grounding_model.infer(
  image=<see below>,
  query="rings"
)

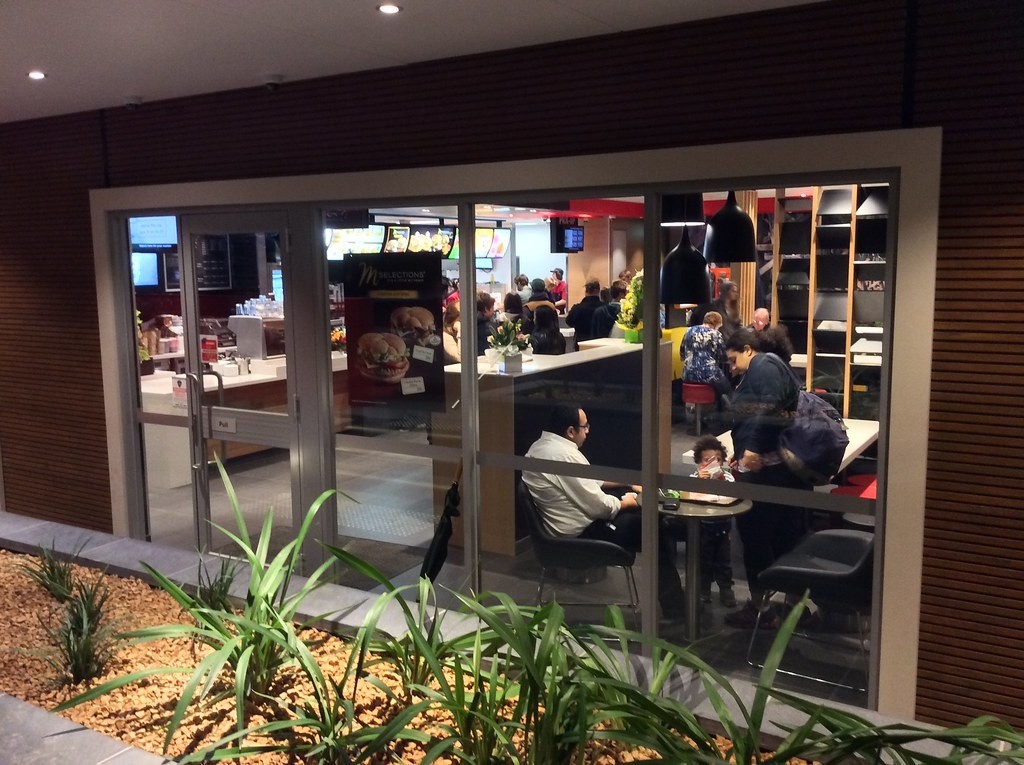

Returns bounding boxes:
[751,460,757,464]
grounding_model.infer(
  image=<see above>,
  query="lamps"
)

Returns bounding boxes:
[661,224,709,304]
[703,189,757,262]
[661,193,705,227]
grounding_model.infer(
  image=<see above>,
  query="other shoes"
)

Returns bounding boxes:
[720,588,739,608]
[698,585,714,601]
[768,598,813,627]
[663,598,705,620]
[725,597,777,630]
[687,427,712,436]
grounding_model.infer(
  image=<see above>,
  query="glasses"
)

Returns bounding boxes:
[752,319,768,325]
[578,423,590,429]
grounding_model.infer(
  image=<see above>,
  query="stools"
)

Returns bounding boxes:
[681,384,716,436]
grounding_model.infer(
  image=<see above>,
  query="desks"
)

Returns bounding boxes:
[636,489,754,644]
[432,338,673,557]
[682,417,880,486]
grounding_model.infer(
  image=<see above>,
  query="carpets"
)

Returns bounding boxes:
[214,523,431,592]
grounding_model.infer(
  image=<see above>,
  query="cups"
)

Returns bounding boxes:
[141,326,179,354]
[235,294,283,318]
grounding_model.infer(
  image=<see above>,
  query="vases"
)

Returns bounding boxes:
[624,331,644,343]
[485,346,534,364]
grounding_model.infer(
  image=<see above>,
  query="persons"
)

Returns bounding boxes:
[521,402,704,620]
[680,280,836,630]
[439,267,632,363]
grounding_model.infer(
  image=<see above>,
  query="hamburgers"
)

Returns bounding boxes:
[357,332,412,384]
[390,305,435,346]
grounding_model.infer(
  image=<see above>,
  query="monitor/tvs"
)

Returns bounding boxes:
[129,215,178,293]
[563,226,584,249]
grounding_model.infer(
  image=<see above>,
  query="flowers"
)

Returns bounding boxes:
[487,318,530,353]
[614,272,644,331]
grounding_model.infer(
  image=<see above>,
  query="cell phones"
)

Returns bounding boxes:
[663,498,680,510]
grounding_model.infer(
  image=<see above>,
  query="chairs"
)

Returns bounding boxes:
[747,467,879,693]
[516,482,640,643]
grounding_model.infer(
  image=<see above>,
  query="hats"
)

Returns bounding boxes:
[529,278,545,292]
[550,268,563,276]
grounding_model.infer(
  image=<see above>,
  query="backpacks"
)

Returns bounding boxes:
[748,350,849,487]
